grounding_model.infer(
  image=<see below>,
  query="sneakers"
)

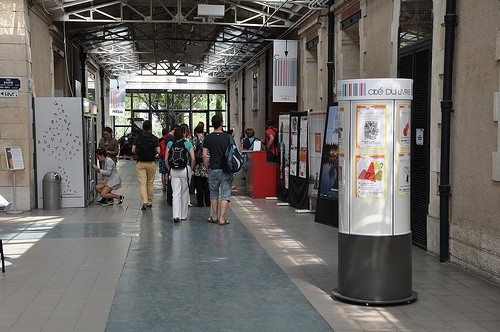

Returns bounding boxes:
[102,199,114,206]
[117,195,124,206]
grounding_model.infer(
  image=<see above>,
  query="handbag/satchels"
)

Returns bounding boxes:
[195,140,203,165]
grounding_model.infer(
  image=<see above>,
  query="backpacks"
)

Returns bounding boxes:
[243,137,256,150]
[138,133,155,161]
[168,139,187,169]
[225,134,244,173]
[272,128,278,145]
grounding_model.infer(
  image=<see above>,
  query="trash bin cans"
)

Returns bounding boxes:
[43,171,61,210]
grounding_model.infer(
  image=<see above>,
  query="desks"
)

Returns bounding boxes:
[246,163,277,199]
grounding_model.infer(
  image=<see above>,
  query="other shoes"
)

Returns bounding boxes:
[141,202,152,210]
[189,203,193,207]
[181,218,187,220]
[174,218,180,223]
[97,198,108,204]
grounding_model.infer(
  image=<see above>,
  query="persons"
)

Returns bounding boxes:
[165,126,196,222]
[131,120,160,211]
[158,117,279,206]
[202,114,237,226]
[92,149,125,206]
[96,127,118,202]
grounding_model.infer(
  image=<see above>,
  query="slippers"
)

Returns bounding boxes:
[219,220,229,225]
[207,216,218,223]
